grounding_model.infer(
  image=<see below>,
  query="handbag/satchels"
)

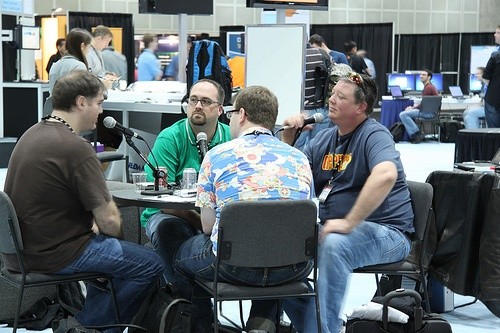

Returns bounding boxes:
[129,281,197,333]
[345,288,452,333]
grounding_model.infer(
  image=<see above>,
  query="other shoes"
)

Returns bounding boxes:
[413,135,424,143]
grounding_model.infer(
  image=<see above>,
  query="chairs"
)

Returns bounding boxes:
[413,96,441,141]
[353,180,434,314]
[45,96,130,183]
[0,191,122,333]
[189,199,322,333]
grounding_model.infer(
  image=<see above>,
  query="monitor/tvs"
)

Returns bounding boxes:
[388,73,415,91]
[389,86,403,97]
[415,73,443,93]
[449,86,463,97]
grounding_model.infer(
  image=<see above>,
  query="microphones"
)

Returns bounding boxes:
[103,116,144,140]
[197,131,208,160]
[277,113,324,132]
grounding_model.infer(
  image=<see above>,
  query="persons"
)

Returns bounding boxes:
[165,42,193,81]
[88,24,119,90]
[100,40,128,90]
[48,28,93,100]
[278,72,414,333]
[462,25,500,130]
[137,32,163,82]
[173,85,317,333]
[45,38,66,81]
[399,68,442,144]
[3,70,164,333]
[139,79,237,274]
[304,34,376,109]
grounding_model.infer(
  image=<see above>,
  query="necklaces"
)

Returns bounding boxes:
[41,114,76,133]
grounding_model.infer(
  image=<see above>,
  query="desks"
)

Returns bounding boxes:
[454,128,500,162]
[437,99,482,118]
[102,101,232,127]
[426,170,500,319]
[112,188,246,331]
[380,99,413,128]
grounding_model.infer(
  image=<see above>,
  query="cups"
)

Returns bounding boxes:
[132,173,147,193]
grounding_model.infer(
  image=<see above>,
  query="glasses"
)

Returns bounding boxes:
[187,97,219,107]
[343,71,368,103]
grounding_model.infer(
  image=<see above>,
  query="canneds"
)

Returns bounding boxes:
[154,167,167,191]
[183,167,197,189]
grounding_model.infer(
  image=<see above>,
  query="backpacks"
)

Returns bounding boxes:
[314,48,353,109]
[388,122,406,143]
[180,33,233,105]
